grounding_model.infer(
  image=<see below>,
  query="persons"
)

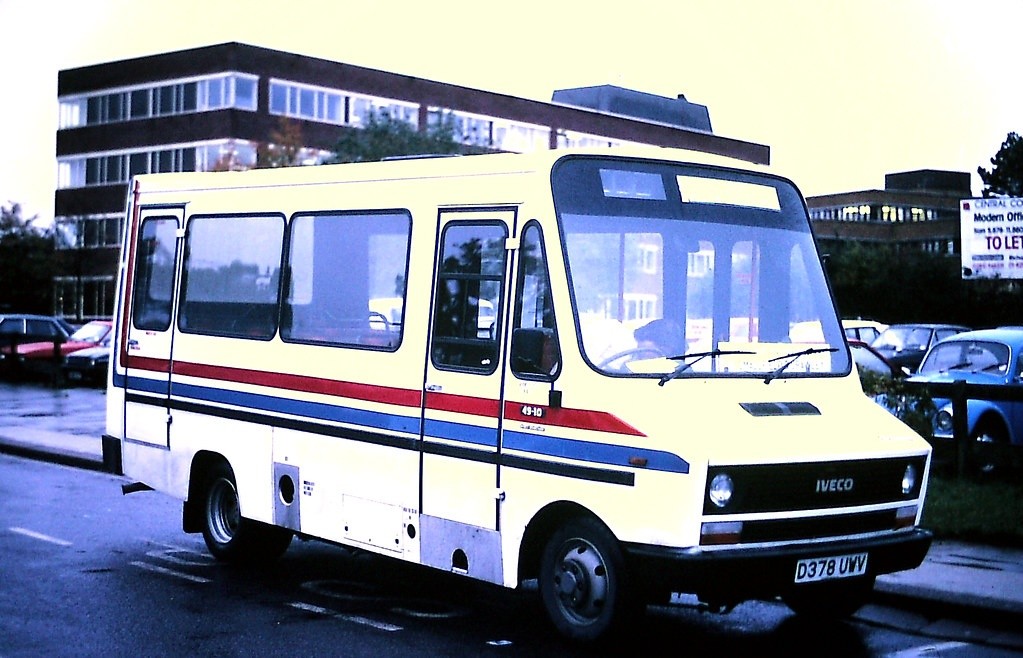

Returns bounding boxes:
[549,314,688,384]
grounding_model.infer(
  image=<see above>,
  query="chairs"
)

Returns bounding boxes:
[228,303,557,375]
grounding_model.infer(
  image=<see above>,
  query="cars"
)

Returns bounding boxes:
[843,339,908,414]
[836,319,884,347]
[66,327,116,369]
[869,323,974,376]
[3,320,113,362]
[1,313,75,340]
[872,326,1023,473]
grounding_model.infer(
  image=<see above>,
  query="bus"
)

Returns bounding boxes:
[101,145,935,658]
